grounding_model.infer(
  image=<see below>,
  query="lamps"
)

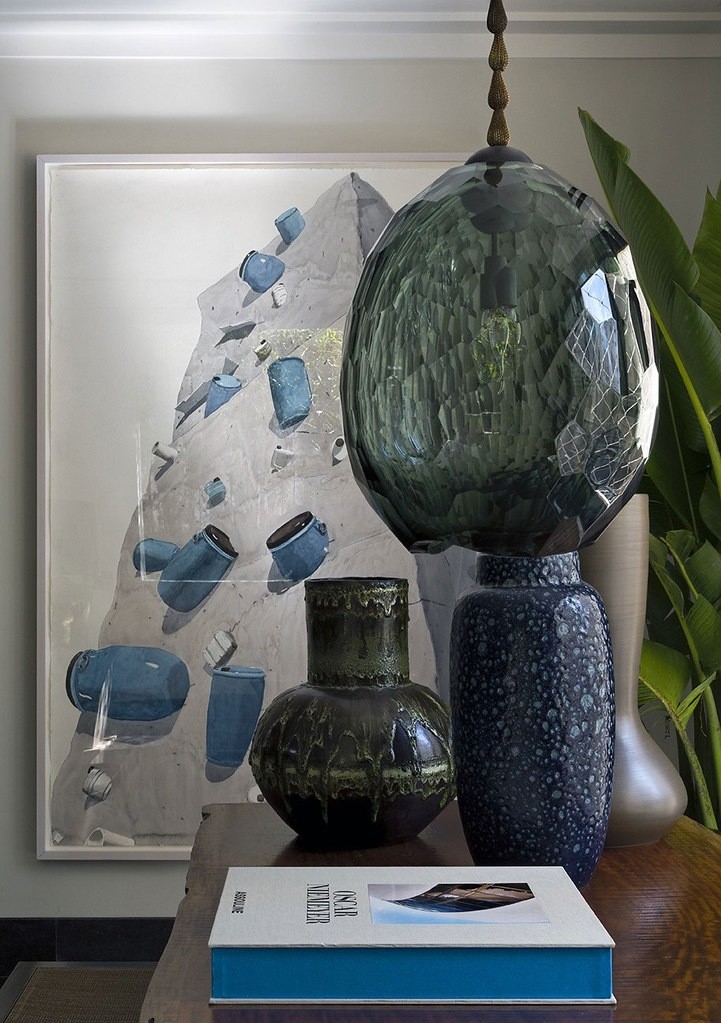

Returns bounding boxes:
[336,0,662,557]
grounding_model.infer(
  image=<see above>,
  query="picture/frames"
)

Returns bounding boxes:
[38,152,485,859]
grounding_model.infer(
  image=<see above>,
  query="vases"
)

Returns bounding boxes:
[579,494,690,851]
[447,552,613,891]
[247,576,460,852]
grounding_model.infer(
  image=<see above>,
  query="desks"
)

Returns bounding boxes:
[139,801,721,1023]
